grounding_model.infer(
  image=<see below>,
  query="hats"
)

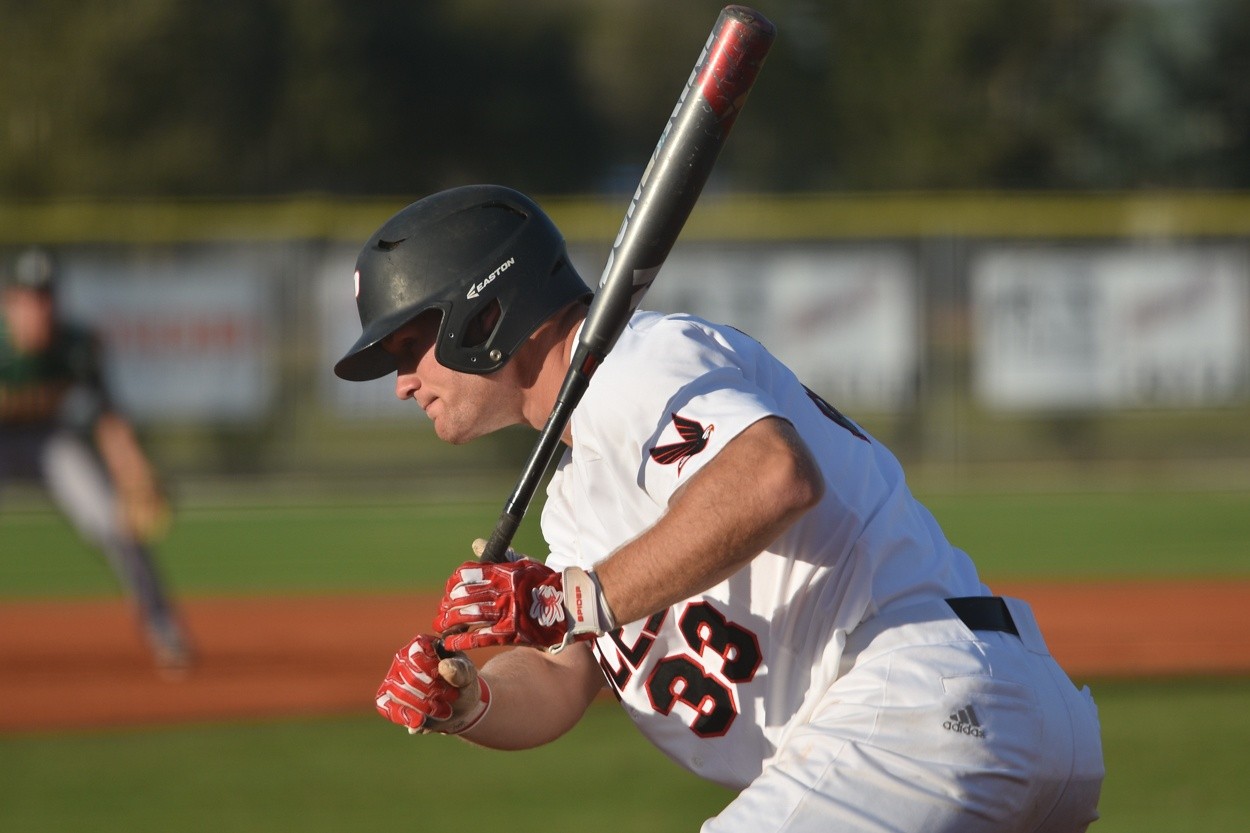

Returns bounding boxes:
[6,243,63,294]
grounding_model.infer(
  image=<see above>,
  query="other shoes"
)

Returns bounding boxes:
[136,629,207,683]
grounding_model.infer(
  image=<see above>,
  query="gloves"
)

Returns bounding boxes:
[432,538,618,655]
[373,634,491,737]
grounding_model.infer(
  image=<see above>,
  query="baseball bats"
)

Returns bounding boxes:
[416,0,776,737]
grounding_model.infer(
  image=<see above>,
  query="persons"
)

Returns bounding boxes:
[1,249,185,659]
[334,184,1102,832]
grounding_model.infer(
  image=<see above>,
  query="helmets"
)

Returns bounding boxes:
[334,185,596,381]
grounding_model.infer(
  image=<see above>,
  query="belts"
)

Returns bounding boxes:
[943,596,1018,636]
